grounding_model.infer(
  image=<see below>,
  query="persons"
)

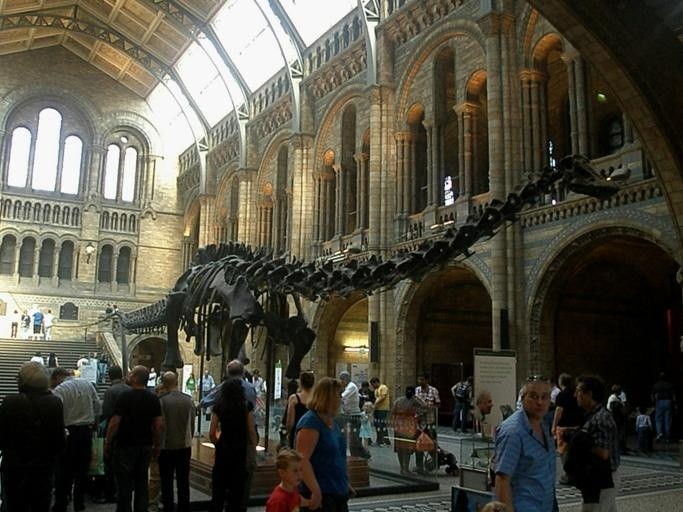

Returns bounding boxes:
[0,361,66,512]
[356,380,375,408]
[606,385,628,455]
[20,309,30,338]
[293,377,356,511]
[196,370,215,421]
[68,347,110,384]
[196,359,258,512]
[92,366,133,506]
[450,376,473,435]
[338,370,360,417]
[246,368,265,398]
[146,385,170,511]
[635,407,652,454]
[156,370,163,386]
[46,351,58,369]
[49,366,101,512]
[360,401,373,450]
[285,373,314,450]
[550,373,583,486]
[31,351,44,364]
[543,377,560,410]
[392,384,429,478]
[474,390,492,435]
[413,371,441,474]
[517,374,533,410]
[265,451,312,512]
[146,367,155,388]
[650,369,675,444]
[370,377,389,447]
[102,365,162,512]
[157,371,196,512]
[44,311,53,340]
[556,375,621,512]
[10,309,18,338]
[32,308,43,341]
[493,380,558,512]
[208,378,257,512]
[183,373,197,399]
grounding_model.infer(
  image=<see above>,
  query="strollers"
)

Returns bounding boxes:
[408,411,460,477]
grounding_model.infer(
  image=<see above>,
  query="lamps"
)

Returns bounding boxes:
[85,242,95,255]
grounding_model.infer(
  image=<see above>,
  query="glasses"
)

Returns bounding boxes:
[525,374,551,385]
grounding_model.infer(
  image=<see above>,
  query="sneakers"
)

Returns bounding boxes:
[379,441,392,449]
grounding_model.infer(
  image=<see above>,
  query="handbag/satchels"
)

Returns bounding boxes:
[415,424,435,453]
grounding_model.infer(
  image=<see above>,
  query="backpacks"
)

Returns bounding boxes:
[454,381,470,401]
